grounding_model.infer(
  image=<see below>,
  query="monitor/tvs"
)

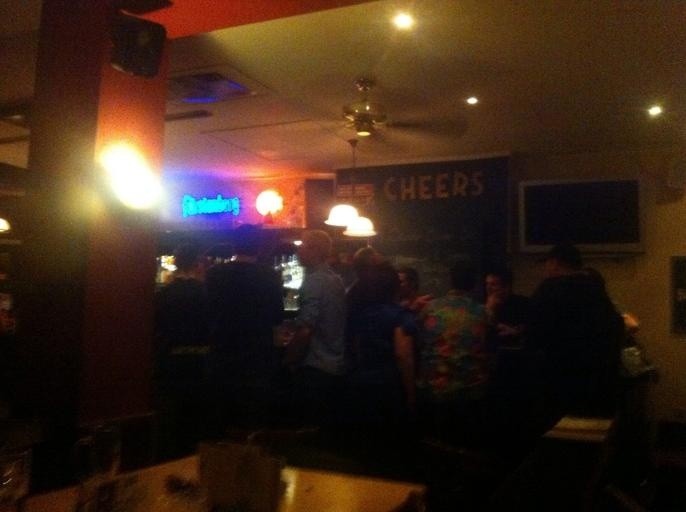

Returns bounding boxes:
[524,179,640,244]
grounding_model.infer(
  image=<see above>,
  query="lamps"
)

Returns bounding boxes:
[324,139,359,227]
[341,79,391,139]
[342,214,379,238]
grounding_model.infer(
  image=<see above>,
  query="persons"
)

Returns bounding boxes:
[274,230,344,454]
[204,223,284,430]
[525,242,624,512]
[585,268,640,335]
[151,236,214,457]
[395,258,520,512]
[398,268,418,313]
[344,273,420,481]
[483,262,529,336]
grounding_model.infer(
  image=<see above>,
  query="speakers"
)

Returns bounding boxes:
[110,16,167,80]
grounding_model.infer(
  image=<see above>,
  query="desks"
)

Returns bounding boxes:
[0,453,423,512]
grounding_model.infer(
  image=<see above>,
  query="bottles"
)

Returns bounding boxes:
[155,254,176,284]
[272,253,300,289]
